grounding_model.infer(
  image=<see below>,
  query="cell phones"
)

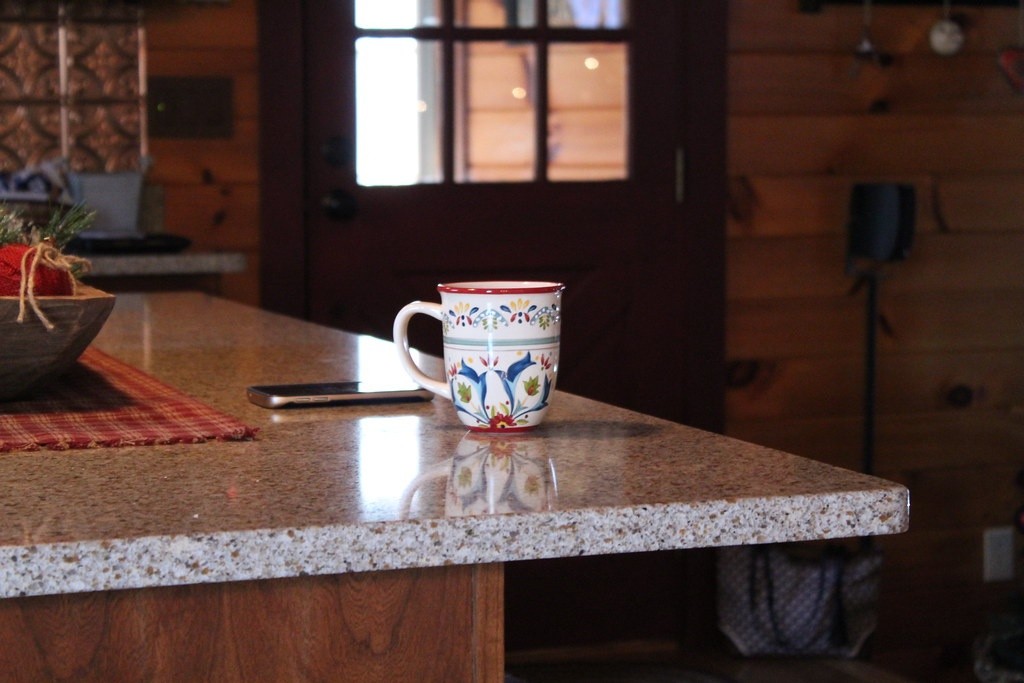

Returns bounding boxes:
[245,379,435,411]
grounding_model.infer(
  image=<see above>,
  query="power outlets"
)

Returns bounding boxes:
[982,526,1017,584]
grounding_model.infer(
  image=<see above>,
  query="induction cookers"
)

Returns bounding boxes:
[66,230,193,258]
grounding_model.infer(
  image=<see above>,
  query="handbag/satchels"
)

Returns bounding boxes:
[707,538,892,661]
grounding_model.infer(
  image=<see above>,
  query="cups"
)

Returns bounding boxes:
[391,276,565,436]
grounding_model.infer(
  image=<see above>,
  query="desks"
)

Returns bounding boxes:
[67,250,250,277]
[1,291,915,679]
